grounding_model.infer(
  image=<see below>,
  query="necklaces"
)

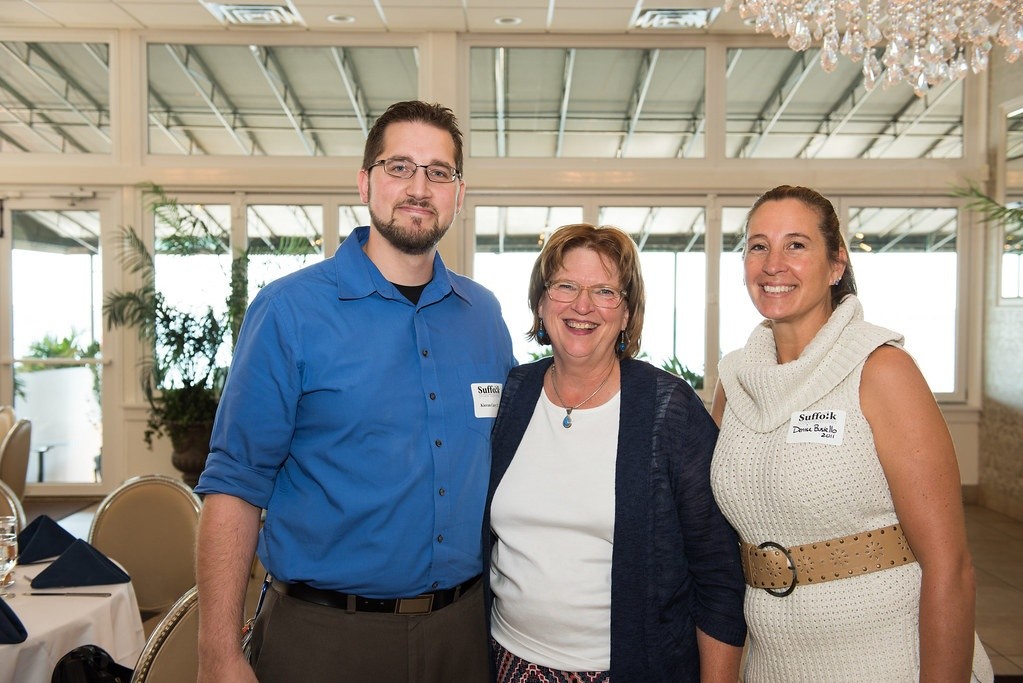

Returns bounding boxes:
[545,353,616,427]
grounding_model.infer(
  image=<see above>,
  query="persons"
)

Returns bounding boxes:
[195,103,526,683]
[710,186,994,683]
[480,223,747,683]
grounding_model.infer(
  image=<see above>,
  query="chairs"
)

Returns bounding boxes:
[131,554,268,683]
[0,419,32,503]
[0,406,16,446]
[86,471,203,613]
[0,480,26,553]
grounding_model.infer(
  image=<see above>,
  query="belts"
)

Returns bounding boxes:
[271,571,486,615]
[738,522,916,597]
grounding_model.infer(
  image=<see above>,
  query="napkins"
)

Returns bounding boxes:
[0,593,28,645]
[17,516,74,565]
[32,538,130,588]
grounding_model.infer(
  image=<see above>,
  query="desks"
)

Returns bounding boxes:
[0,556,144,683]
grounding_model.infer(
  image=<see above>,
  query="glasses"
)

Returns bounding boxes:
[544,280,627,309]
[367,159,461,183]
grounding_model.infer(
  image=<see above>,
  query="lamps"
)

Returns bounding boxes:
[742,0,1023,97]
[201,1,307,30]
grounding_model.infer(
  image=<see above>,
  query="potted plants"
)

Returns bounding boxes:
[109,254,253,488]
